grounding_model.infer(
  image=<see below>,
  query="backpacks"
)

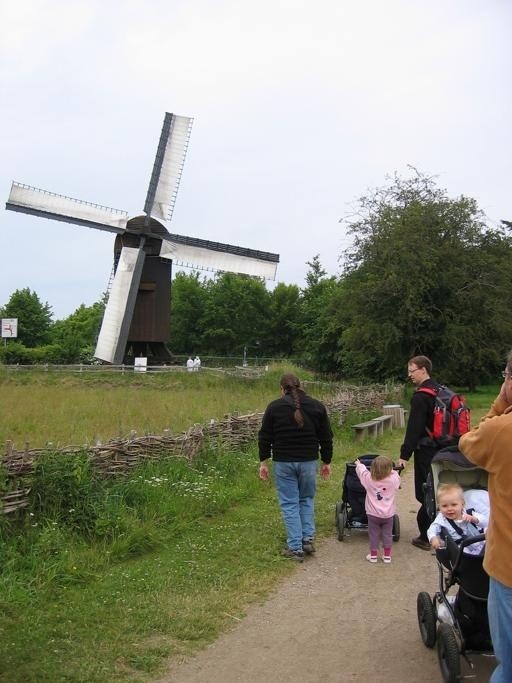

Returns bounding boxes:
[414,384,471,449]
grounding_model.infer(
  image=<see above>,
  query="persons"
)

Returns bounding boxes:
[460,349,512,683]
[399,354,456,552]
[194,355,202,371]
[424,482,488,557]
[353,456,402,567]
[253,373,335,562]
[186,356,195,372]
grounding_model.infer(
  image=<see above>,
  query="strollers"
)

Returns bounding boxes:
[335,455,404,542]
[416,446,498,682]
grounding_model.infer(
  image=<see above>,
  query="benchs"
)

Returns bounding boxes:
[350,419,378,441]
[372,414,395,435]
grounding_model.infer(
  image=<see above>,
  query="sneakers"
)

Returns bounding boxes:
[412,536,431,551]
[366,553,378,562]
[302,536,317,552]
[381,554,391,563]
[281,548,305,561]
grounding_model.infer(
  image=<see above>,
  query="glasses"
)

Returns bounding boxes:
[501,370,512,377]
